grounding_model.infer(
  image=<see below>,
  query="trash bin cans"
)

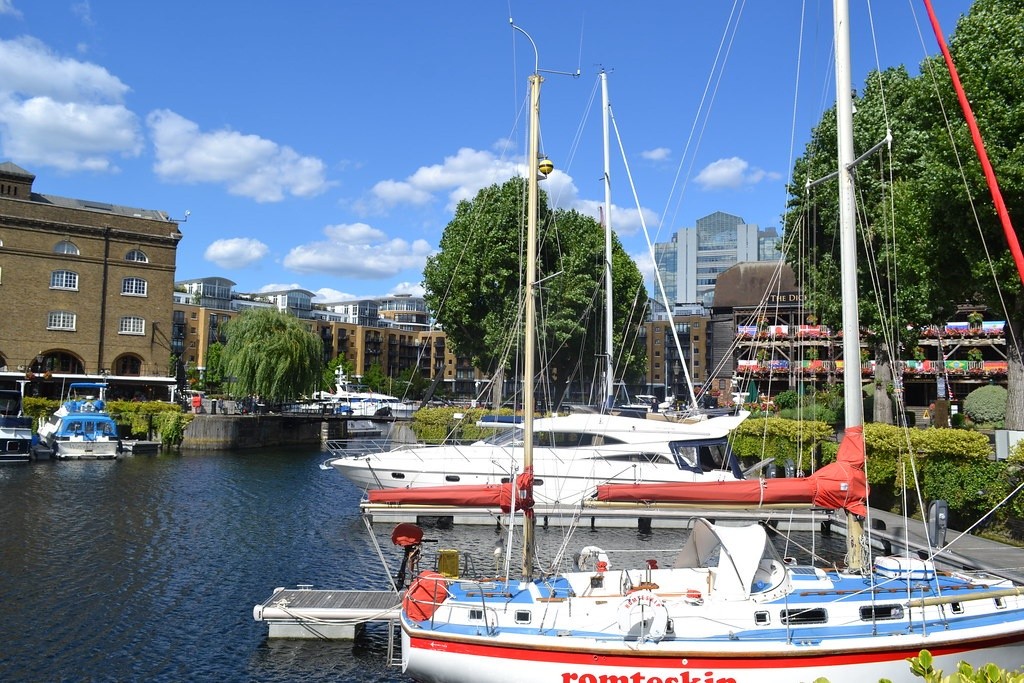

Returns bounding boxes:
[211,399,217,415]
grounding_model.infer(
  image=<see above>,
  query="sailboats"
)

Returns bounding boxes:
[363,1,1024,682]
[317,60,757,498]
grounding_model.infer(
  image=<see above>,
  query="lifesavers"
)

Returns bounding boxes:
[578,545,611,571]
[616,590,670,642]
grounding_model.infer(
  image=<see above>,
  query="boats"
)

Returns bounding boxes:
[281,364,420,422]
[0,390,33,465]
[38,383,120,458]
